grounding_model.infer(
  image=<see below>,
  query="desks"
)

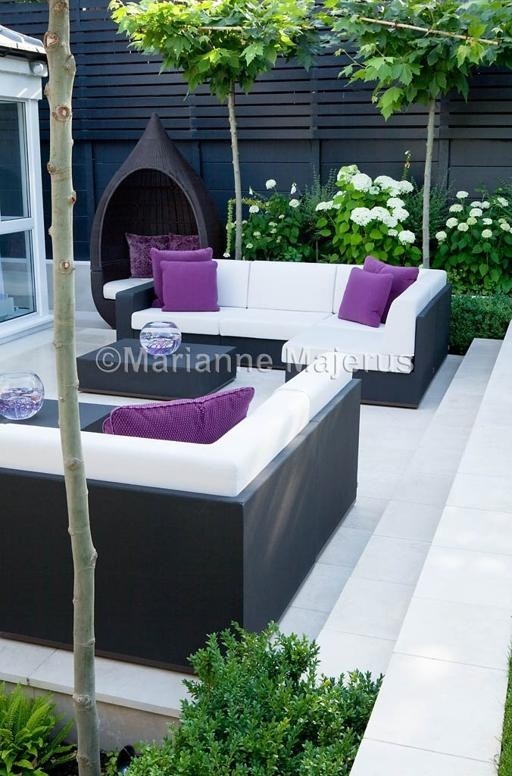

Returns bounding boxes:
[0,397,123,435]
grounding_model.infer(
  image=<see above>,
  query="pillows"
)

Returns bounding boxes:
[166,231,201,255]
[160,259,220,314]
[99,383,264,450]
[150,245,215,308]
[336,267,397,331]
[360,255,422,326]
[123,230,169,279]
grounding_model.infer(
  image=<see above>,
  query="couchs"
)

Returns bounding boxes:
[101,256,452,412]
[0,352,363,685]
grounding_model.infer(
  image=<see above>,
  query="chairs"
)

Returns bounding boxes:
[88,109,226,332]
